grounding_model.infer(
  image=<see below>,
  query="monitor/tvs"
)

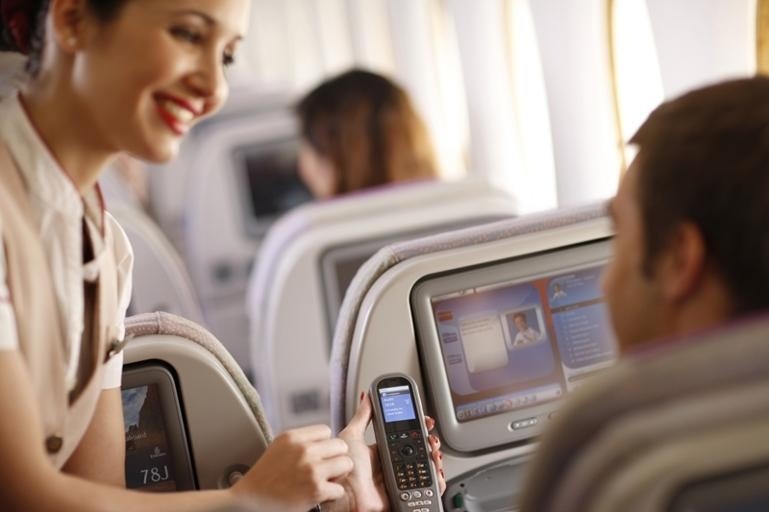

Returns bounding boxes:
[413,237,622,452]
[322,242,380,339]
[232,140,314,236]
[121,366,196,493]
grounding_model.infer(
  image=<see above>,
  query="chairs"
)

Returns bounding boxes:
[99,110,522,438]
[331,191,620,511]
[122,311,320,512]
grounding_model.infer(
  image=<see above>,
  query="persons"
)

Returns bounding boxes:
[512,312,541,348]
[553,283,566,298]
[605,77,769,353]
[0,0,447,512]
[294,70,436,202]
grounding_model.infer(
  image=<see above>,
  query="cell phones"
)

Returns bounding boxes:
[369,372,444,512]
